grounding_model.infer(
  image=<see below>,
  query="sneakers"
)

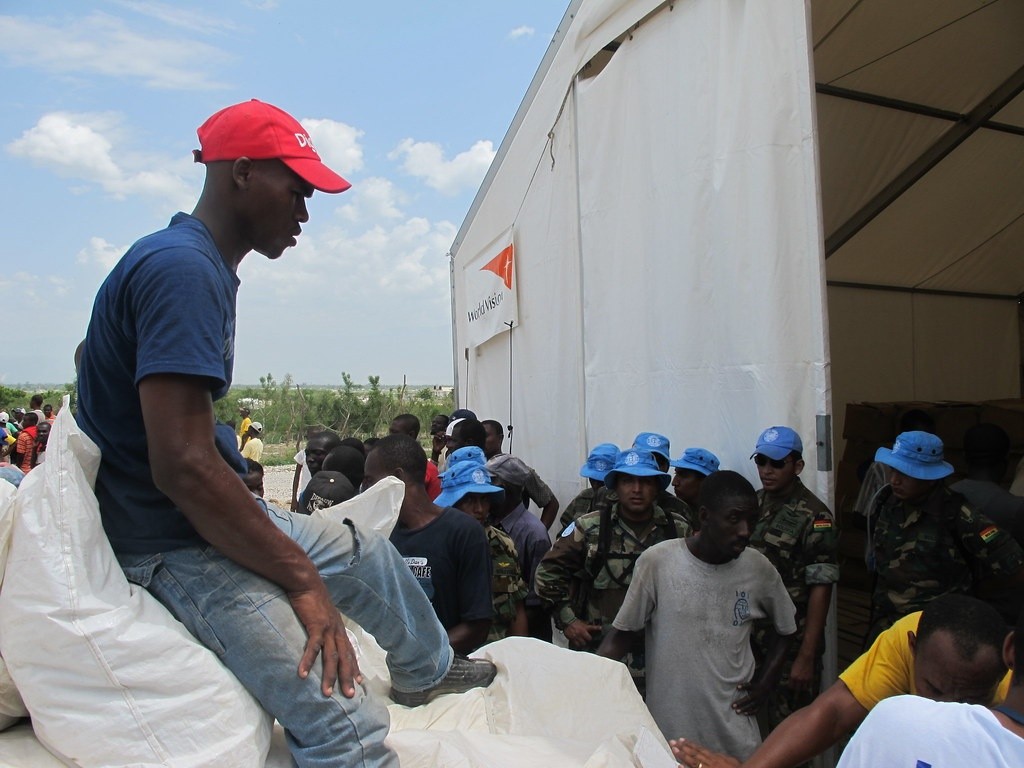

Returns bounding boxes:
[392,657,495,707]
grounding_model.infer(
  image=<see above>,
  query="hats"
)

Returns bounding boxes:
[477,454,531,487]
[12,408,27,415]
[432,459,506,507]
[443,418,468,436]
[0,412,9,424]
[632,432,671,461]
[239,406,250,414]
[580,443,621,482]
[604,449,671,493]
[750,425,802,462]
[874,432,954,480]
[437,445,488,478]
[670,447,721,477]
[450,409,476,423]
[248,421,263,433]
[193,98,353,193]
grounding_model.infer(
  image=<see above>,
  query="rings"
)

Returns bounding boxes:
[699,763,702,768]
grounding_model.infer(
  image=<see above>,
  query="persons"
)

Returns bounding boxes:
[227,407,265,497]
[0,393,57,490]
[289,410,1024,768]
[75,98,496,768]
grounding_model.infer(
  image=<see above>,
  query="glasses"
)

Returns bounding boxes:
[753,455,796,469]
